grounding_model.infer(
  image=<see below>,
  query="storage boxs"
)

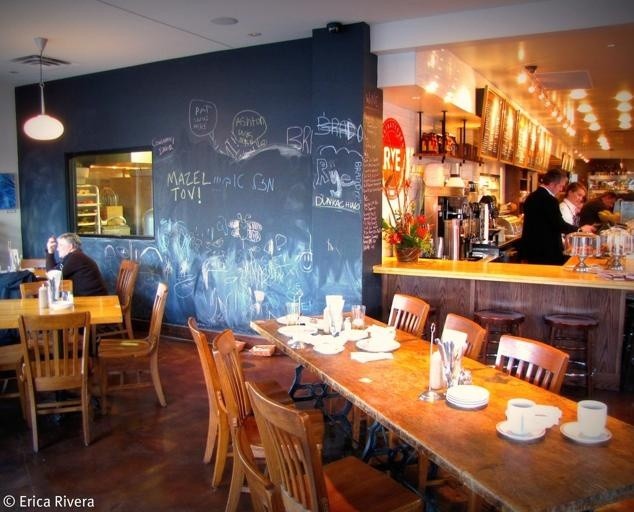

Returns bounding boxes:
[101,205,123,220]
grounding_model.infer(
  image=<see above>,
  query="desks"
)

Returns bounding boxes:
[0,294,123,370]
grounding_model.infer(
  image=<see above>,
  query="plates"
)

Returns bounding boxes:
[276,326,345,355]
[557,421,614,447]
[495,422,546,443]
[340,330,369,342]
[443,386,489,410]
[356,338,402,354]
[277,314,313,323]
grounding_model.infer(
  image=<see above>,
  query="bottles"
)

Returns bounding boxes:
[39,284,49,310]
[420,130,458,157]
[461,370,471,386]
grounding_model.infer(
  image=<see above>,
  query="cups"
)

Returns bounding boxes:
[428,352,442,391]
[505,396,539,435]
[450,217,460,260]
[9,247,20,273]
[432,236,444,258]
[47,268,62,304]
[350,303,367,329]
[576,398,609,438]
[463,142,479,161]
[287,302,302,325]
[497,226,506,242]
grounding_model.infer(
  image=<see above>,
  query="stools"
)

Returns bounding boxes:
[544,312,599,402]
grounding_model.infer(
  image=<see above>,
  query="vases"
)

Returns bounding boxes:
[396,244,420,262]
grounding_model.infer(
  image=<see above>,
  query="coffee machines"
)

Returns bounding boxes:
[424,195,500,258]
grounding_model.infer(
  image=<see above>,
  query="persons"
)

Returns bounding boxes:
[45,232,116,335]
[517,168,597,265]
[559,182,586,245]
[579,191,617,226]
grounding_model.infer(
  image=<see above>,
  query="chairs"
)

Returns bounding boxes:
[187,258,569,511]
[18,311,90,454]
[20,280,74,299]
[92,259,139,355]
[0,344,26,418]
[97,282,168,416]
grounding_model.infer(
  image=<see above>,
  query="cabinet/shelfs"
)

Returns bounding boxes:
[75,184,100,235]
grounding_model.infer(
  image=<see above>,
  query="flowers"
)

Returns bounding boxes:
[382,201,434,255]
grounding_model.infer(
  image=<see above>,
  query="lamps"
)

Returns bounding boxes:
[22,38,65,141]
